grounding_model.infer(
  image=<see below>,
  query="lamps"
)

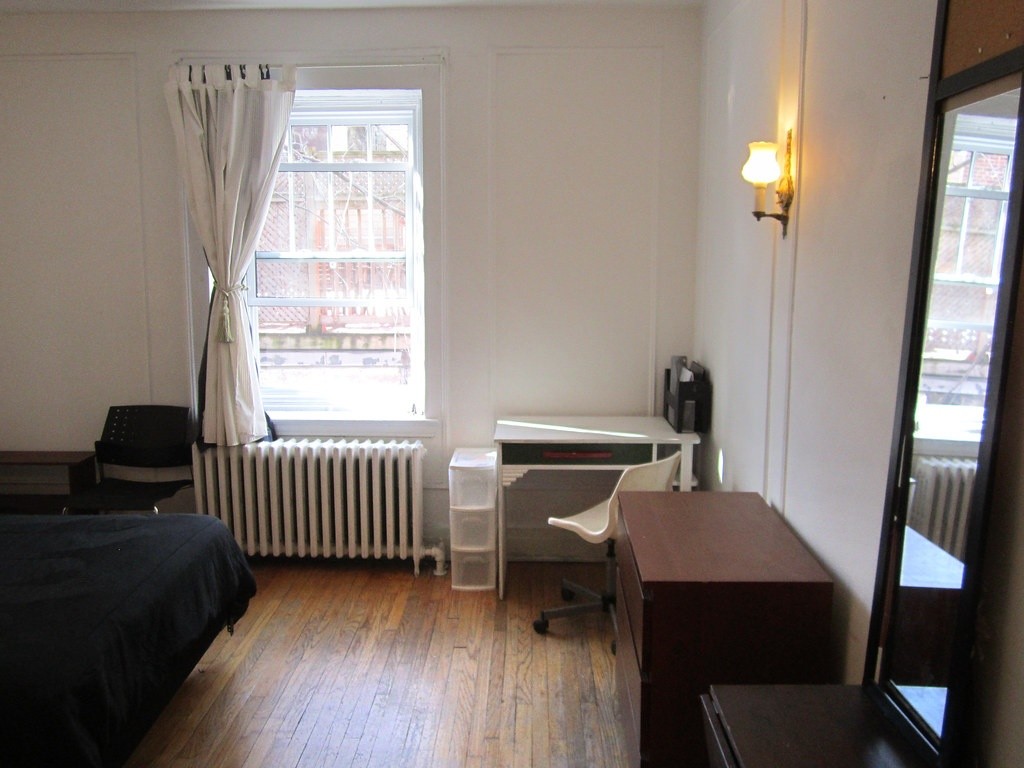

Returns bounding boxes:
[741,129,793,237]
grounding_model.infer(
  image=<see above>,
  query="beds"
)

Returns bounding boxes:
[0,507,259,768]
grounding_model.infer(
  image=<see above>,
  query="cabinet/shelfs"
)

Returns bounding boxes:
[613,489,834,768]
[890,525,965,687]
[449,447,497,592]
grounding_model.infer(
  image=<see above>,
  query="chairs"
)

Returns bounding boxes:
[62,405,194,517]
[535,451,682,655]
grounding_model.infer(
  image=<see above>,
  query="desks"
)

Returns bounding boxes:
[0,451,96,514]
[493,414,700,600]
[700,681,931,768]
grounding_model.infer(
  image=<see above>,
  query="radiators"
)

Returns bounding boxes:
[915,456,979,558]
[192,441,446,577]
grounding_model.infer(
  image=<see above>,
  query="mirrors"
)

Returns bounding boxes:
[857,0,1024,768]
